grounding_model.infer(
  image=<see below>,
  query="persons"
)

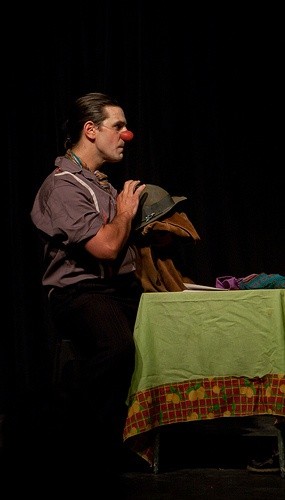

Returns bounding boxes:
[29,91,200,467]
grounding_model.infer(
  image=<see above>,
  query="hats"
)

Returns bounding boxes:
[132,185,187,231]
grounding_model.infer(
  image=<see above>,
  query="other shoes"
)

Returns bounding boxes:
[246,448,285,472]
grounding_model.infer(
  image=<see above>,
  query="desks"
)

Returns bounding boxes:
[123,289,285,478]
